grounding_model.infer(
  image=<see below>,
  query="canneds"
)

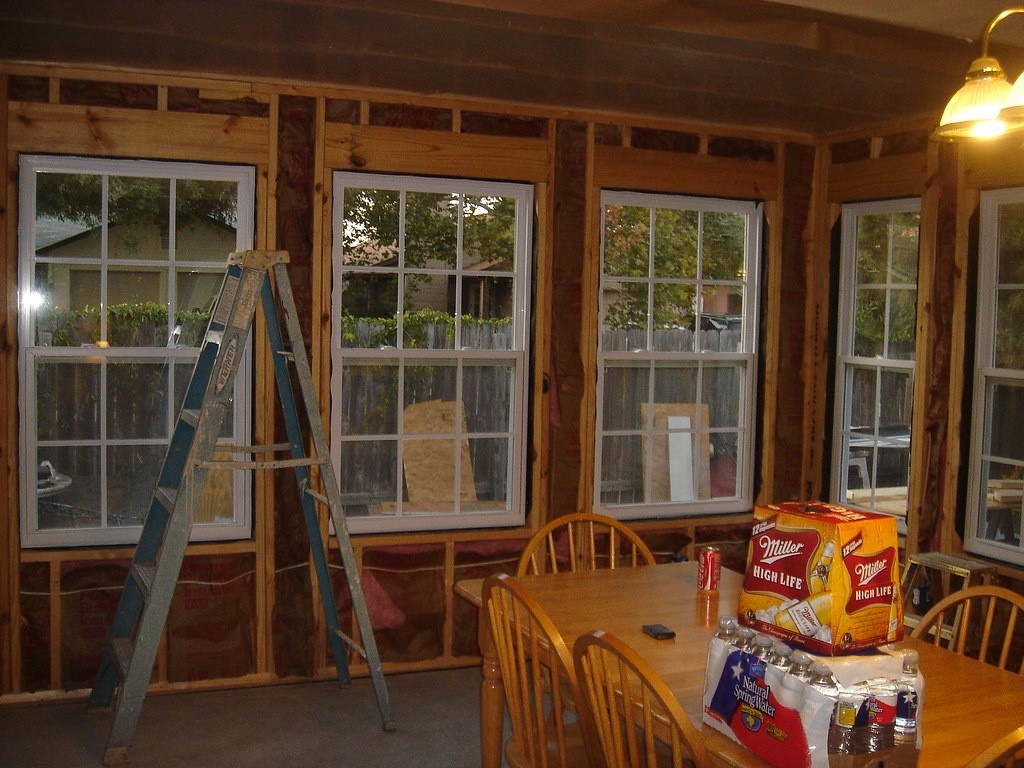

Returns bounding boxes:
[697,546,721,591]
[696,589,720,629]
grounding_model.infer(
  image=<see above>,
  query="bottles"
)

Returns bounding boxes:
[869,677,898,751]
[887,585,899,641]
[782,652,811,692]
[766,645,792,673]
[893,649,921,744]
[809,540,834,596]
[735,627,755,654]
[805,661,839,752]
[774,591,832,637]
[837,683,869,755]
[715,616,735,644]
[752,635,773,662]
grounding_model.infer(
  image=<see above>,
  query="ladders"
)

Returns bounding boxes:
[87,250,399,768]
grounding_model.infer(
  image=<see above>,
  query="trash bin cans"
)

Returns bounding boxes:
[37,467,72,530]
[691,313,742,332]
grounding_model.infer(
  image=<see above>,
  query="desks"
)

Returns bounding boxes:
[452,561,1024,768]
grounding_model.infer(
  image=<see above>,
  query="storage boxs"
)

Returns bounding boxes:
[736,500,903,655]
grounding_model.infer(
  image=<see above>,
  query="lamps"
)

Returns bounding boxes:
[928,5,1024,143]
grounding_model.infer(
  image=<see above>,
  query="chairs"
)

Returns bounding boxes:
[481,573,693,768]
[573,629,715,768]
[910,584,1024,677]
[964,726,1024,768]
[518,512,657,727]
[850,449,872,489]
